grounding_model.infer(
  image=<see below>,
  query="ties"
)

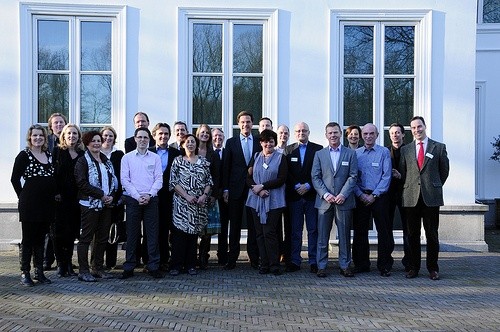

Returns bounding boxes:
[215,148,222,160]
[244,138,251,165]
[417,141,425,169]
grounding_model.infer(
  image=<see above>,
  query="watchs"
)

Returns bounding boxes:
[372,194,377,198]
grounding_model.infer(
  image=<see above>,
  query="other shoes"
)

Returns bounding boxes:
[218,258,226,266]
[148,270,163,279]
[118,269,134,279]
[286,262,301,272]
[316,269,327,278]
[250,259,270,275]
[169,268,180,276]
[310,263,318,273]
[91,268,115,279]
[78,272,97,282]
[41,257,55,269]
[222,261,236,270]
[187,267,198,276]
[340,267,354,278]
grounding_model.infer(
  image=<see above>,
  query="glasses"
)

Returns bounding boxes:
[136,136,149,140]
[295,129,308,133]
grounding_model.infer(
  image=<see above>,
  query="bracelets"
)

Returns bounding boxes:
[148,194,154,198]
[202,192,208,197]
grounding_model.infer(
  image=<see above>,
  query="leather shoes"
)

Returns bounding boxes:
[272,268,284,276]
[19,270,35,287]
[33,267,51,284]
[429,270,440,280]
[403,267,418,279]
[380,264,391,277]
[351,265,363,274]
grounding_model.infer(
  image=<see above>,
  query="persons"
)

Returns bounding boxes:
[392,117,449,280]
[311,122,358,277]
[118,127,164,280]
[10,111,409,288]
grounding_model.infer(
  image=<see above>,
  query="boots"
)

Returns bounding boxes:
[54,241,78,279]
